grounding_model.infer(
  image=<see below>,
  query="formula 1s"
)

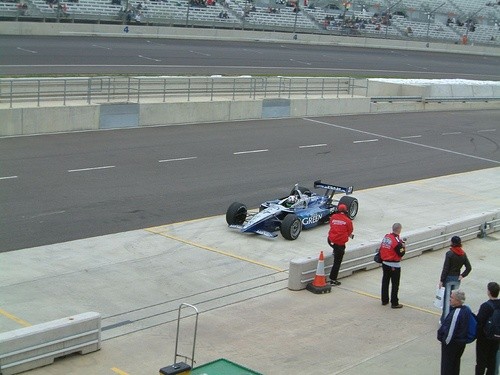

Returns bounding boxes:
[226,180,358,241]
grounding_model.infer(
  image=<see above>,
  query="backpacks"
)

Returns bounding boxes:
[462,305,477,344]
[486,301,500,334]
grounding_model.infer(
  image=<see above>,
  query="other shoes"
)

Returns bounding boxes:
[391,304,403,308]
[381,301,389,305]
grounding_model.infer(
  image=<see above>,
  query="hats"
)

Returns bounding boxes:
[338,204,347,212]
[452,236,461,244]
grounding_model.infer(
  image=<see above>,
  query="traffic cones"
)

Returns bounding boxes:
[311,251,328,287]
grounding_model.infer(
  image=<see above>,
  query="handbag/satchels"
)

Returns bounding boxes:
[374,252,383,263]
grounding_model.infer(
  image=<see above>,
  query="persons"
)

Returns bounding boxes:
[138,3,148,12]
[475,281,500,375]
[218,10,229,18]
[323,13,367,28]
[16,2,28,15]
[436,289,471,375]
[57,3,66,13]
[446,17,476,32]
[439,235,472,325]
[267,6,280,14]
[110,0,120,4]
[189,0,217,8]
[327,204,353,286]
[404,25,407,34]
[343,1,351,10]
[118,5,141,25]
[370,8,391,30]
[379,223,405,308]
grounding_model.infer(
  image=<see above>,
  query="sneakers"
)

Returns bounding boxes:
[329,279,342,285]
